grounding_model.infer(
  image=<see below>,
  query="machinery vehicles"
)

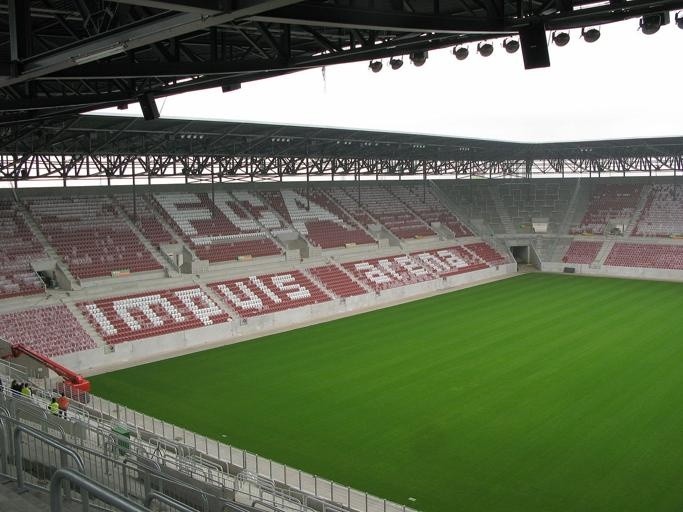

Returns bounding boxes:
[0,341,89,405]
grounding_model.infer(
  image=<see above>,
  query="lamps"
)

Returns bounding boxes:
[366,7,682,72]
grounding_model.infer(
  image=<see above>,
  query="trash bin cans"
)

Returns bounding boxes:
[111,427,129,456]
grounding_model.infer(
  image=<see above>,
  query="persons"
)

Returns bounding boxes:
[48,398,58,416]
[10,380,31,399]
[58,393,68,420]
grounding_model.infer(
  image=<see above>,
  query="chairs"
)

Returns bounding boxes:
[515,177,683,275]
[0,182,513,360]
